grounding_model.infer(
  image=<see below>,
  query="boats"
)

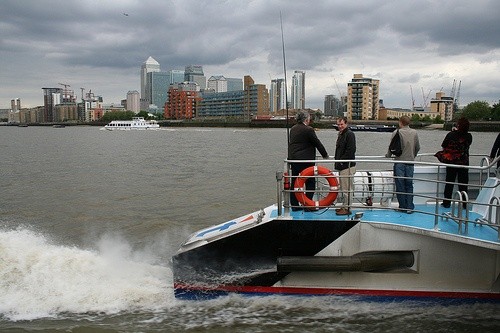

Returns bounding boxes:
[331,124,398,132]
[170,153,500,308]
[53,125,65,128]
[104,117,160,131]
[19,125,28,127]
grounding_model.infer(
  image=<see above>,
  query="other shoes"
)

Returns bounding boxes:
[395,208,406,212]
[407,209,412,214]
[304,208,319,212]
[335,208,352,215]
[292,207,303,211]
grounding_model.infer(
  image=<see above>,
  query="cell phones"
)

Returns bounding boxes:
[452,127,456,131]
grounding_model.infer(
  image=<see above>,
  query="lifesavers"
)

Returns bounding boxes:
[294,166,339,210]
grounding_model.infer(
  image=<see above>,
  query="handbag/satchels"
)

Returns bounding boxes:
[389,128,402,157]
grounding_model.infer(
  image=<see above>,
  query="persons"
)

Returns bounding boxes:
[385,115,420,214]
[288,110,329,212]
[335,116,357,215]
[441,118,472,208]
[490,132,500,179]
